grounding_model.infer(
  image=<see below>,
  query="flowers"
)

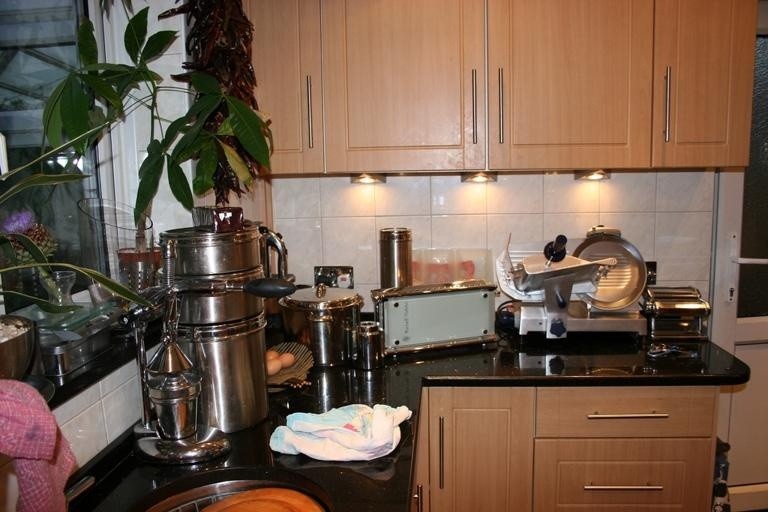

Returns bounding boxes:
[3,209,59,270]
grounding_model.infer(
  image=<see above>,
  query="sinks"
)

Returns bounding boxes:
[130,466,333,512]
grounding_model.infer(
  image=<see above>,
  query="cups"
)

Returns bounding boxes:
[38,270,77,308]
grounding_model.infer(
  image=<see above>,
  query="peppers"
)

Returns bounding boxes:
[175,0,258,206]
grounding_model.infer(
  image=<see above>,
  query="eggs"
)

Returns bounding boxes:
[267,358,281,375]
[280,352,295,368]
[265,351,279,361]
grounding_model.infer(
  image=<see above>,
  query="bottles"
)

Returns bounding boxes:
[355,319,386,371]
[379,225,413,289]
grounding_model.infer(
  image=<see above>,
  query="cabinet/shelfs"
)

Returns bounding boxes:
[425,387,535,511]
[652,2,757,172]
[318,1,653,177]
[239,2,325,178]
[533,387,719,512]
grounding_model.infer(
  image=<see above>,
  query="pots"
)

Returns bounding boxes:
[154,220,296,438]
[278,282,366,369]
